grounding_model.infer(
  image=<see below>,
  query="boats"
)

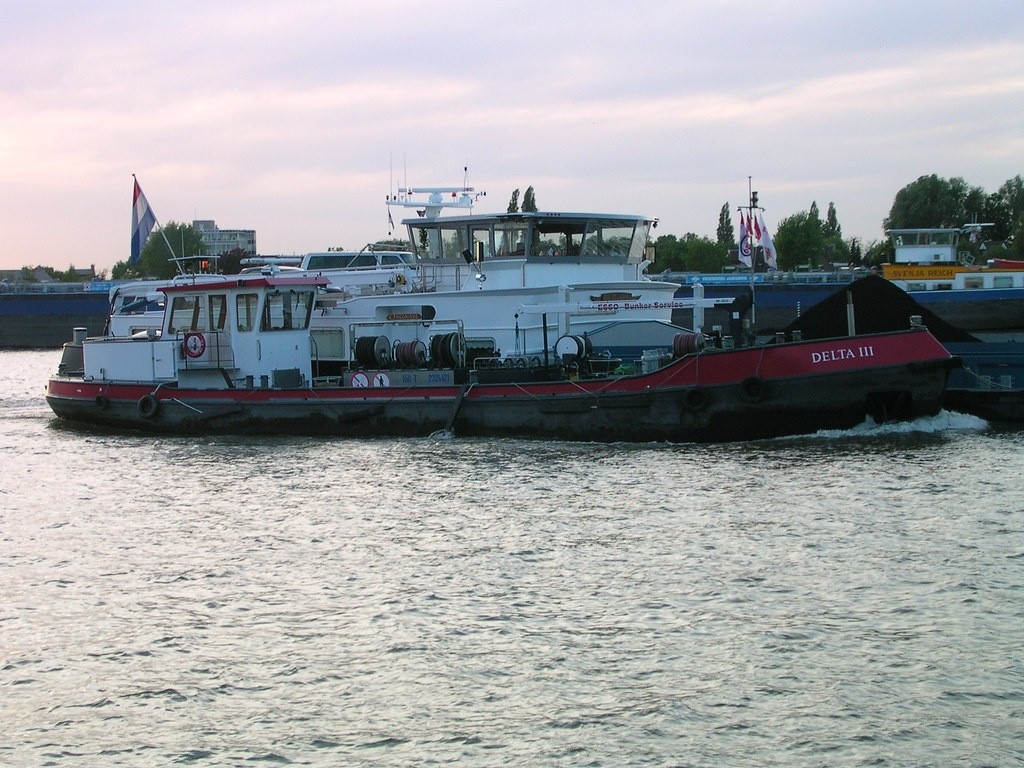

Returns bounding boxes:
[44,171,963,443]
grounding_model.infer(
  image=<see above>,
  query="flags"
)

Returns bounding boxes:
[388,209,394,231]
[739,207,778,270]
[131,173,156,270]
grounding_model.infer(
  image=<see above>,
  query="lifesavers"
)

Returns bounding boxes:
[137,394,160,419]
[95,394,108,411]
[183,332,206,358]
